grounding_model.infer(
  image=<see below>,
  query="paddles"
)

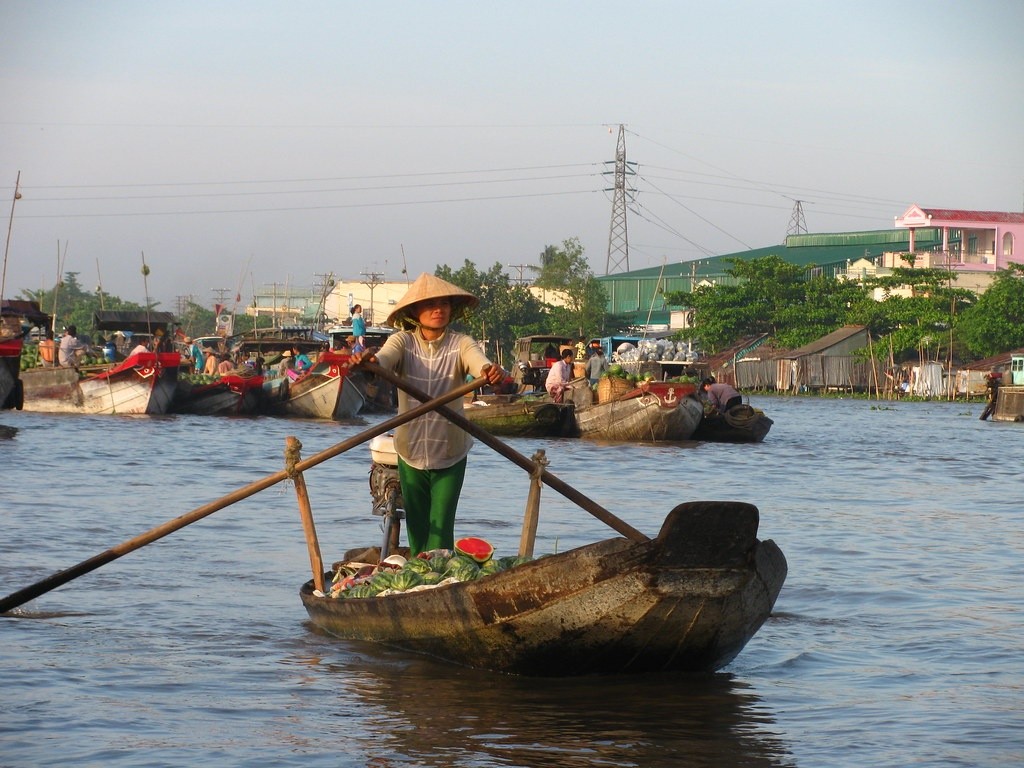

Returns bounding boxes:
[0,362,493,614]
[347,346,648,541]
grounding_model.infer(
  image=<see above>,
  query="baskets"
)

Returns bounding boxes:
[598,376,634,405]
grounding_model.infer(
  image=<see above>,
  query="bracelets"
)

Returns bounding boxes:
[300,367,302,369]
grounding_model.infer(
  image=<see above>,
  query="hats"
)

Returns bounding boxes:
[184,337,192,342]
[387,272,480,330]
[282,351,291,356]
[202,346,219,355]
[345,336,356,341]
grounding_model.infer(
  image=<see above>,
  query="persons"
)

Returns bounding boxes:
[278,350,294,377]
[567,339,598,360]
[349,303,366,352]
[545,349,573,404]
[184,337,204,375]
[585,347,609,386]
[39,325,116,367]
[345,336,362,354]
[129,335,160,355]
[702,376,742,415]
[292,343,312,371]
[317,342,334,360]
[202,346,266,376]
[347,272,506,559]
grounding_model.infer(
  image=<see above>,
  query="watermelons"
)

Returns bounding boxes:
[704,406,711,413]
[591,364,653,391]
[90,355,111,364]
[345,538,555,599]
[188,373,221,385]
[20,344,36,371]
[679,375,698,384]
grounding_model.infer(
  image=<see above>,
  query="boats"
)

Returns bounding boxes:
[510,335,704,440]
[695,404,775,444]
[15,353,180,414]
[300,434,787,672]
[172,375,264,417]
[462,383,575,438]
[262,351,367,419]
